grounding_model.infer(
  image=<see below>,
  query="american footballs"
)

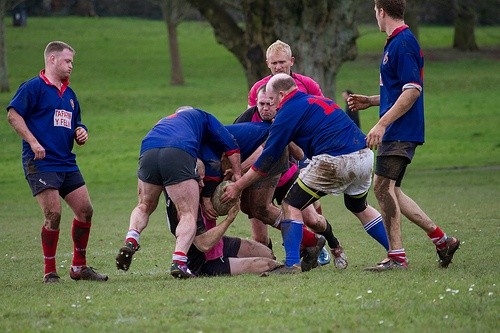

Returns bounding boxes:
[212,181,240,216]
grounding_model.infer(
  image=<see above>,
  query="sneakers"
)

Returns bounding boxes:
[365,257,408,271]
[318,247,330,265]
[301,234,325,271]
[115,241,135,271]
[43,272,61,283]
[260,265,300,277]
[436,235,460,267]
[70,266,108,281]
[329,240,348,270]
[170,263,196,278]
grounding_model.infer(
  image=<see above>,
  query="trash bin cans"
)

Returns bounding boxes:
[13,6,26,26]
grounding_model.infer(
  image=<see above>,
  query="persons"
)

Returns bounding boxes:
[248,40,329,265]
[6,40,109,283]
[163,158,281,277]
[342,89,360,127]
[347,0,461,271]
[199,123,325,272]
[116,105,242,281]
[234,83,347,270]
[219,72,410,264]
